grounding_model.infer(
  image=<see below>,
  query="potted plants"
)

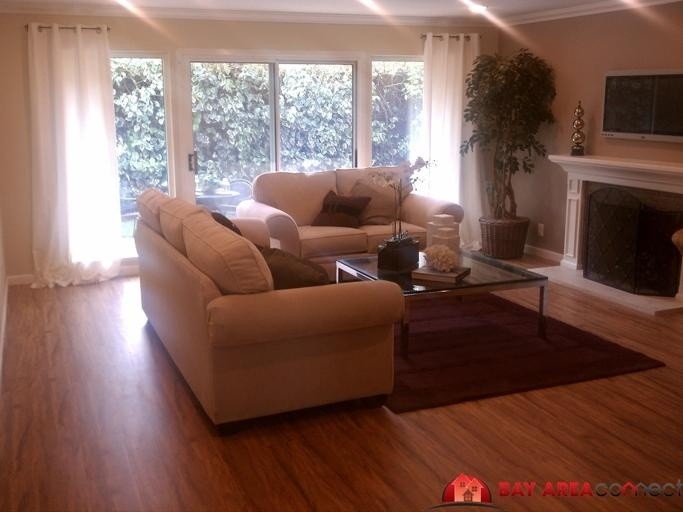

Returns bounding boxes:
[459,48,556,261]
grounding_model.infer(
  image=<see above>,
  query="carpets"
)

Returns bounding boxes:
[382,292,665,415]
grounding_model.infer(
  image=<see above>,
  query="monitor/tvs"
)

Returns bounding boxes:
[599,68,683,144]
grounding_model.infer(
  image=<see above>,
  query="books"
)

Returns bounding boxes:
[412,264,470,283]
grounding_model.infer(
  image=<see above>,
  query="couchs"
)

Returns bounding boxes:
[237,167,465,284]
[135,189,407,436]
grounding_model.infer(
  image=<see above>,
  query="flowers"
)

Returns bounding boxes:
[365,156,434,241]
[424,245,459,273]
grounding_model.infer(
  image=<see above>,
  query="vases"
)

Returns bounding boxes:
[377,234,419,286]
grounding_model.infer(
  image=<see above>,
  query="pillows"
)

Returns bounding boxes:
[350,179,397,225]
[312,190,372,228]
[260,247,329,289]
[210,212,263,252]
